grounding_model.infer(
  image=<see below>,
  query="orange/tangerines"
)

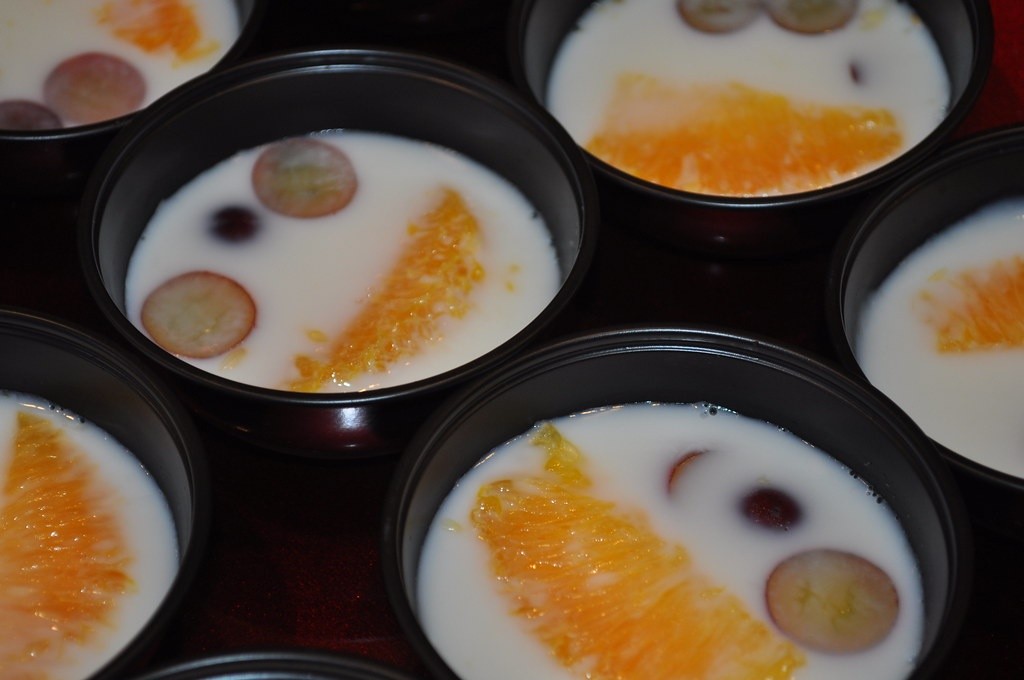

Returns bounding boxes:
[294,187,484,390]
[577,78,895,200]
[468,424,809,680]
[923,254,1024,352]
[94,0,215,64]
[0,411,141,680]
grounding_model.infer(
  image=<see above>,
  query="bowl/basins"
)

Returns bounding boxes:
[137,647,412,680]
[1,308,223,679]
[79,44,597,509]
[0,0,259,195]
[375,320,960,679]
[823,122,1023,501]
[514,0,993,251]
